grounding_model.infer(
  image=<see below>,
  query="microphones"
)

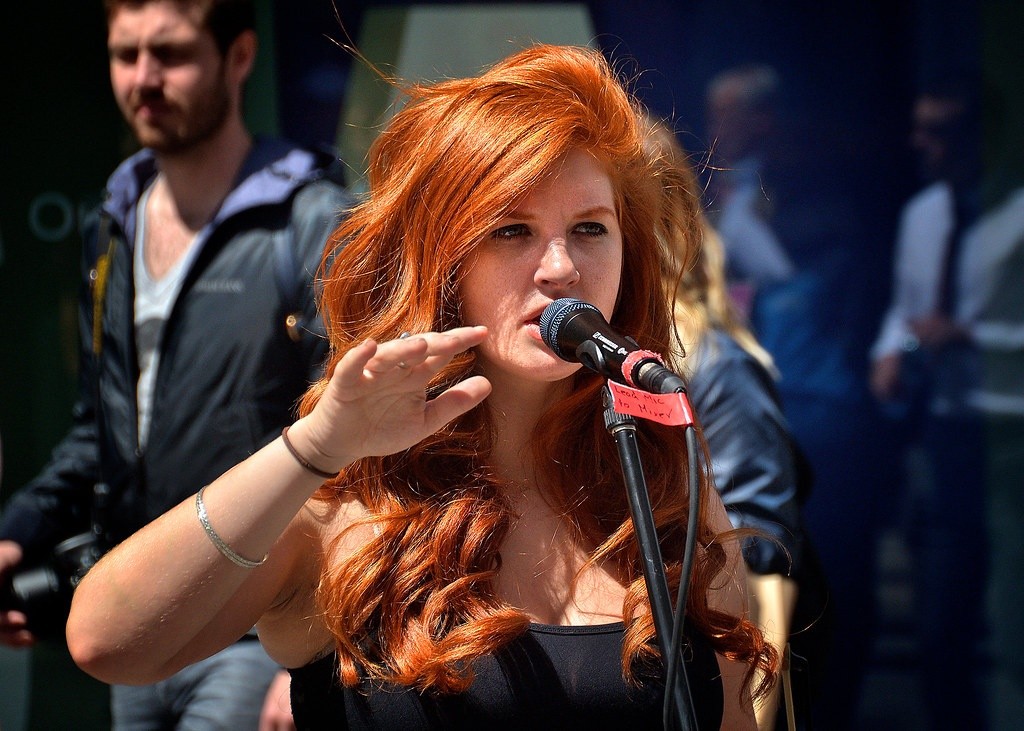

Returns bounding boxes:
[540,298,687,398]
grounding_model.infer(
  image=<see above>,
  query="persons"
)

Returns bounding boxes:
[0,0,1024,731]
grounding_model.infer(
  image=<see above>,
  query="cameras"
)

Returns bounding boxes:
[5,525,112,640]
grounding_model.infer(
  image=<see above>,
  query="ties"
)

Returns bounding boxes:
[935,185,976,373]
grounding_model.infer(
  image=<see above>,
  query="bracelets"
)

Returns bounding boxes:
[197,485,269,569]
[279,426,341,480]
